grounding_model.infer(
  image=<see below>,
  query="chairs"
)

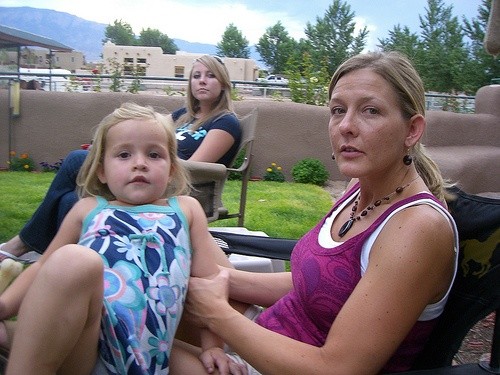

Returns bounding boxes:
[186,109,259,227]
[209,186,500,375]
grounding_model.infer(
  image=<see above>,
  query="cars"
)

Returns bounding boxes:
[255,74,289,87]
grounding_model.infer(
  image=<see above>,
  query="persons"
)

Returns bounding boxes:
[21,80,41,90]
[0,103,249,375]
[0,52,458,375]
[0,55,244,264]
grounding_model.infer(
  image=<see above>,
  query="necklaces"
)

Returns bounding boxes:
[338,176,420,237]
